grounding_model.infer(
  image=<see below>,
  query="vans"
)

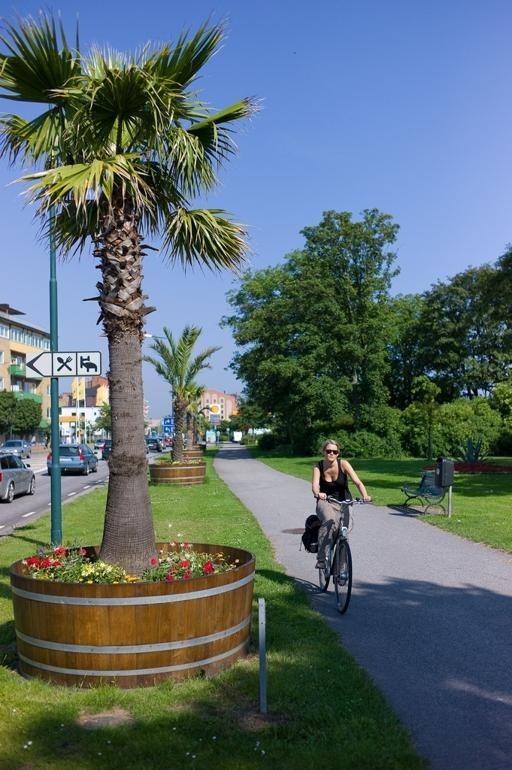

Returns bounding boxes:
[0,452,35,504]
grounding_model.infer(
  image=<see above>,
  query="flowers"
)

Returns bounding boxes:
[20,540,243,585]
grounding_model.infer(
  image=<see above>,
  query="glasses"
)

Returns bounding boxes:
[323,448,339,455]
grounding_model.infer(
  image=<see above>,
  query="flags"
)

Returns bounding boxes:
[78,378,84,400]
[72,378,77,400]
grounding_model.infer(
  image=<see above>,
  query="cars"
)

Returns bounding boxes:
[0,439,32,459]
[46,443,98,476]
[94,439,105,449]
[146,435,173,453]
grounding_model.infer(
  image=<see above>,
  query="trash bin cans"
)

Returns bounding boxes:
[202,445,206,452]
[422,466,439,474]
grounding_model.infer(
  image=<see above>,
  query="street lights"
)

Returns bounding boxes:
[141,333,175,417]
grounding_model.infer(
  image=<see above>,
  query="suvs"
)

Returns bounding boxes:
[101,439,112,460]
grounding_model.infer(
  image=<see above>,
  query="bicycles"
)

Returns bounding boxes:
[312,494,372,614]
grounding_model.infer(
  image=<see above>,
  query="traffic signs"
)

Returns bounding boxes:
[23,348,101,380]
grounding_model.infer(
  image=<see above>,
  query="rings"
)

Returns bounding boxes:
[322,495,325,497]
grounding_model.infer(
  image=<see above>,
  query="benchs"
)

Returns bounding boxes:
[400,470,452,515]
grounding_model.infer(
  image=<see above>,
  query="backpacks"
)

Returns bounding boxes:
[299,514,323,554]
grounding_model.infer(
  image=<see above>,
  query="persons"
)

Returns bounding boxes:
[311,439,371,586]
